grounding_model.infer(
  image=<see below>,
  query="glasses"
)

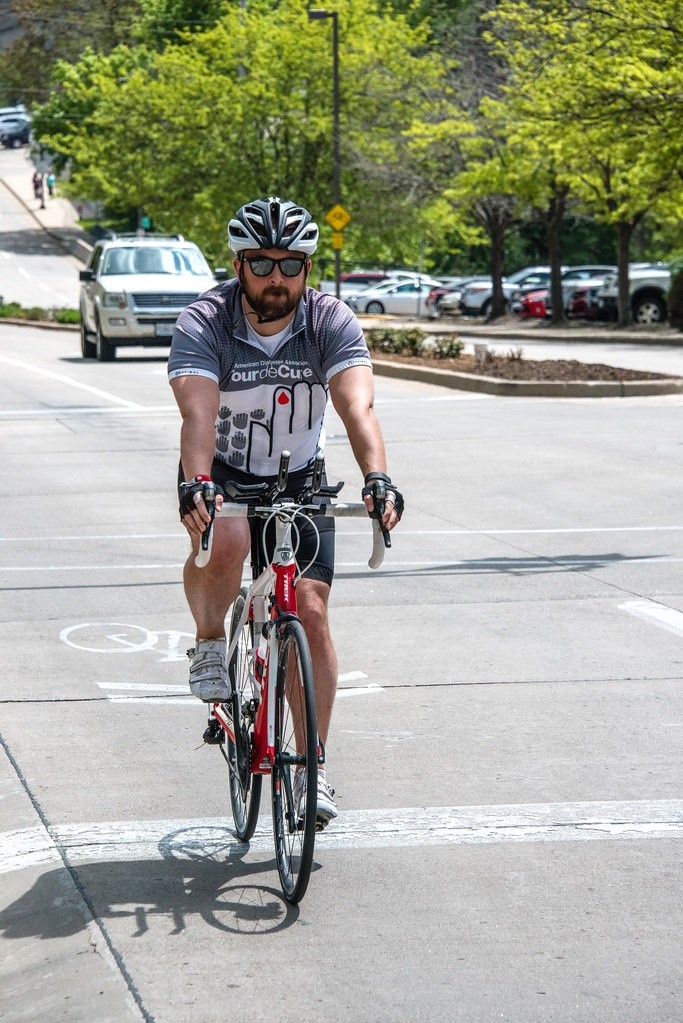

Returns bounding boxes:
[242,256,305,277]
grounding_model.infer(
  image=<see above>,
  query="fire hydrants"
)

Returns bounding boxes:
[33,173,43,200]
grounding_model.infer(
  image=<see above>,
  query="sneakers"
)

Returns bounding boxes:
[188,636,232,701]
[293,768,338,820]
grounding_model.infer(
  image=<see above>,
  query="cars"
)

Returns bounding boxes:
[319,260,683,334]
[0,106,35,148]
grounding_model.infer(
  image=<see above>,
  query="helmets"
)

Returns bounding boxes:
[227,196,319,255]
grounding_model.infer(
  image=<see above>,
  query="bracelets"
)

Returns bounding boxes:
[365,472,391,486]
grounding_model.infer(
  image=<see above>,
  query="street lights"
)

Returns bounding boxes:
[306,7,342,302]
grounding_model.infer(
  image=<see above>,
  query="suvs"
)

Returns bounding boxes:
[79,234,228,361]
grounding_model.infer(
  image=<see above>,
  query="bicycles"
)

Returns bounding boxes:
[195,450,392,908]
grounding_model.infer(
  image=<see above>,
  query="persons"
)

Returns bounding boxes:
[46,173,55,195]
[166,195,403,819]
[34,173,42,198]
